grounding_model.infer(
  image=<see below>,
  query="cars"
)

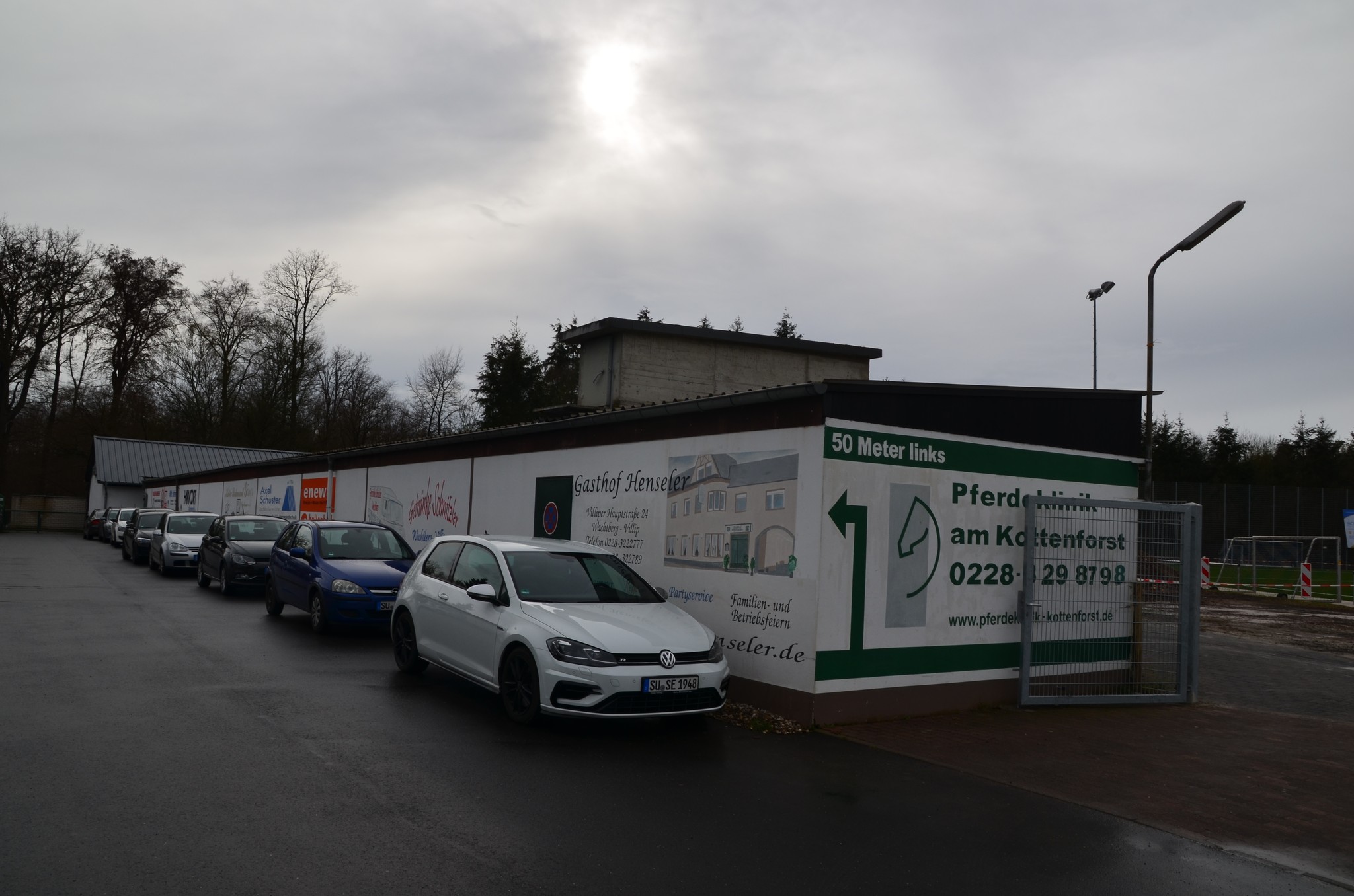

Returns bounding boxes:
[110,507,142,548]
[264,518,425,636]
[388,533,731,728]
[121,507,177,564]
[196,515,298,597]
[83,509,108,541]
[148,511,221,577]
[98,506,124,543]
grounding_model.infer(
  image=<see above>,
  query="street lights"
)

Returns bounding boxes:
[1143,197,1247,574]
[1085,281,1116,392]
